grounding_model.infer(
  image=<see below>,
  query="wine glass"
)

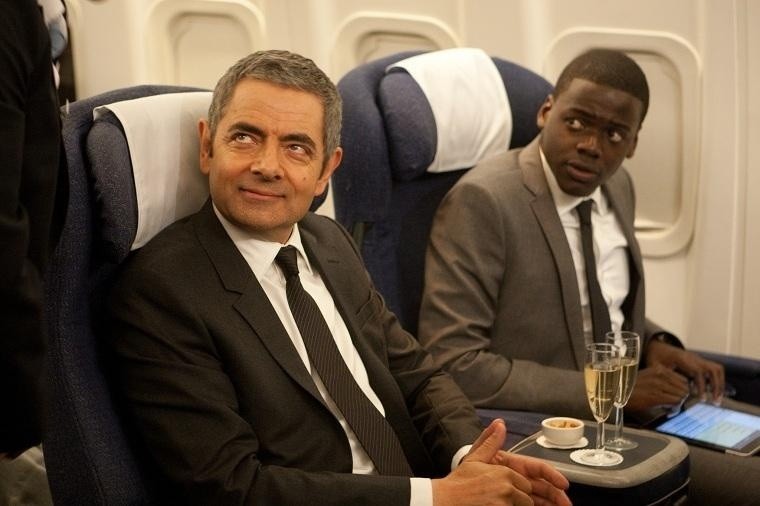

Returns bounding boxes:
[579,339,620,465]
[604,330,639,451]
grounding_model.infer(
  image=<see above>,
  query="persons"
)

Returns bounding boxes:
[416,49,760,506]
[1,0,70,460]
[103,49,570,506]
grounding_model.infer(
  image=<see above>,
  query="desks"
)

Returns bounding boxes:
[506,415,693,506]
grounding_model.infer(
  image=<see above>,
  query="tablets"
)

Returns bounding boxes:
[642,394,760,457]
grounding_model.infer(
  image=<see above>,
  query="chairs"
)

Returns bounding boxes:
[328,47,759,437]
[30,84,528,506]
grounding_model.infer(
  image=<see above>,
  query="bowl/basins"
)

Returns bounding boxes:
[539,415,585,446]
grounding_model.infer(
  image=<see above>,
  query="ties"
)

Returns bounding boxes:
[577,197,615,357]
[275,244,412,477]
